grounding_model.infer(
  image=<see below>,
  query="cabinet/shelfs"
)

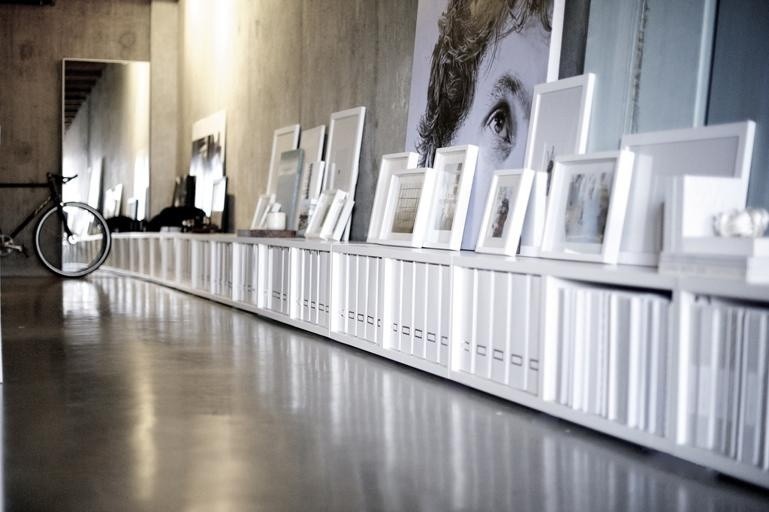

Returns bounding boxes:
[63,229,153,280]
[153,232,769,489]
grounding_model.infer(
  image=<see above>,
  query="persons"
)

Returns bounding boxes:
[492,190,509,238]
[414,1,552,251]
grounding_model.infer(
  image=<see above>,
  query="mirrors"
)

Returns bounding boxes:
[57,57,153,276]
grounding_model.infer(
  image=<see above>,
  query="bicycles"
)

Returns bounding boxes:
[1,171,113,279]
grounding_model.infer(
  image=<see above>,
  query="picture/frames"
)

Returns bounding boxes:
[160,71,769,286]
[80,155,149,232]
